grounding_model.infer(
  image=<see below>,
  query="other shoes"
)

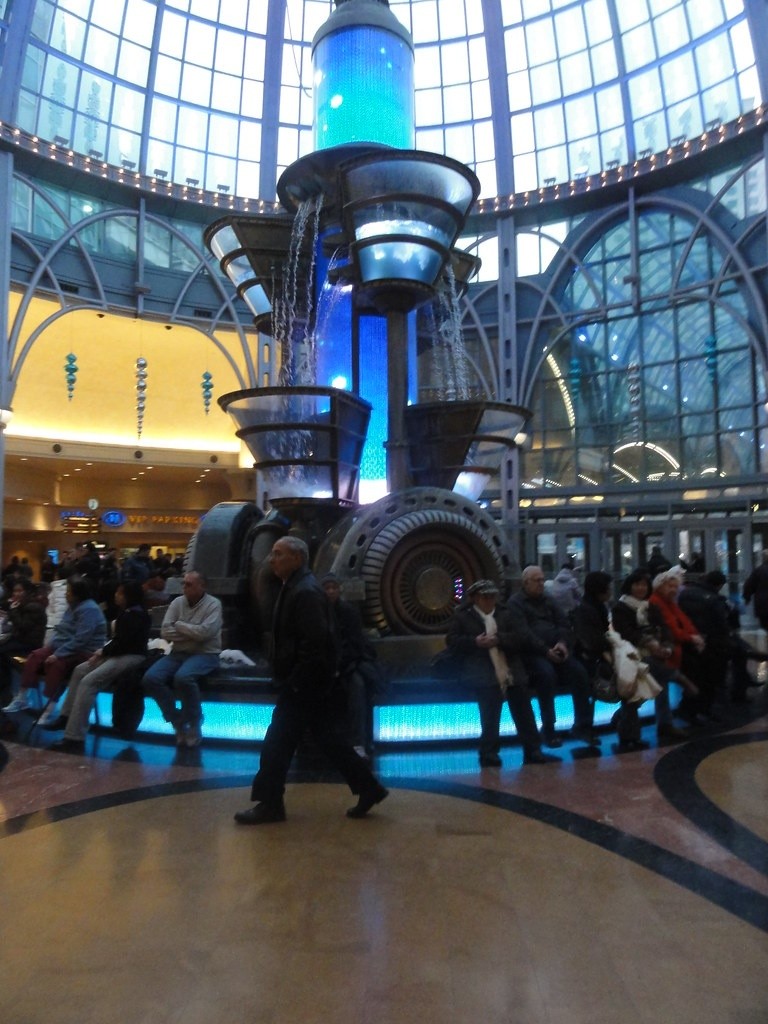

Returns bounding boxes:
[479,752,501,769]
[52,738,86,751]
[540,725,563,748]
[185,720,202,747]
[567,725,603,745]
[234,798,288,825]
[524,750,565,763]
[621,738,649,747]
[47,715,67,730]
[172,709,185,746]
[347,777,389,820]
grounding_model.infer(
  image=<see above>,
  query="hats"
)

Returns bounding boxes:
[320,573,341,587]
[466,578,499,595]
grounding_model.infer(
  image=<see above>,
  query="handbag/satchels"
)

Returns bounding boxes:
[590,667,620,703]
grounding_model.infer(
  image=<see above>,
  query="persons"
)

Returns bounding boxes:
[446,546,768,767]
[232,537,390,824]
[0,541,224,752]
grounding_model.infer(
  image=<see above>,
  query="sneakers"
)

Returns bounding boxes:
[35,713,56,727]
[3,696,30,712]
